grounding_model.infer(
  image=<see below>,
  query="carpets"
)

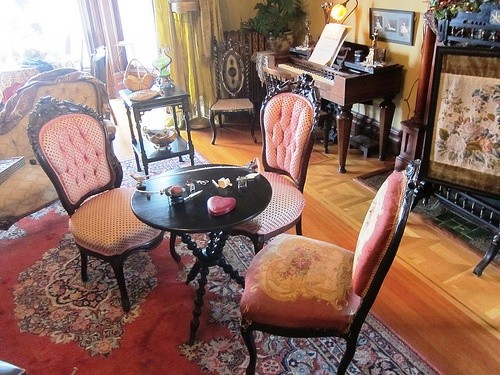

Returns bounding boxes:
[0,144,439,375]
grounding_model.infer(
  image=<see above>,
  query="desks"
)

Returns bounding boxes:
[132,163,273,348]
[0,66,40,105]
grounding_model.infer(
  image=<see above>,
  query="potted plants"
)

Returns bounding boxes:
[247,0,306,51]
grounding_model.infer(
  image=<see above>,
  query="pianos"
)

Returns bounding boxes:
[254,45,406,173]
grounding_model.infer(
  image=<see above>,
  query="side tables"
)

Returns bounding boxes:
[118,84,195,175]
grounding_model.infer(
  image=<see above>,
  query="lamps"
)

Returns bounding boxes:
[331,0,359,24]
[169,0,210,129]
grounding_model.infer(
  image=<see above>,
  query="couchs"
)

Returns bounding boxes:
[0,67,116,219]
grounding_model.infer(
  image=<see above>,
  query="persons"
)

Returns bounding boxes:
[371,29,379,48]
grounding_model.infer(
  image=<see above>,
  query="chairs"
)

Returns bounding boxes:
[229,73,321,254]
[27,95,180,313]
[207,37,258,145]
[234,158,422,375]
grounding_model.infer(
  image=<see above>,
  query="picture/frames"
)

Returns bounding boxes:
[368,7,415,46]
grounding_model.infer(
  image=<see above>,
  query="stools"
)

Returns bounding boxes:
[319,110,330,154]
